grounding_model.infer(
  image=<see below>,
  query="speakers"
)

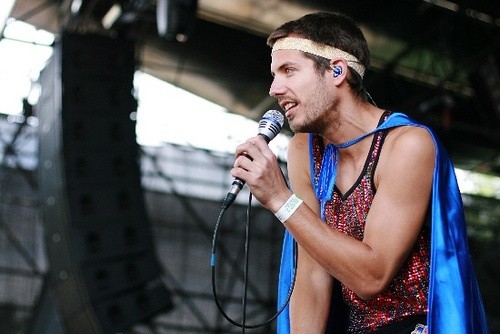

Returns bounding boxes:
[38,44,176,334]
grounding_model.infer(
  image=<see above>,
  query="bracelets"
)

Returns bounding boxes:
[274,194,303,223]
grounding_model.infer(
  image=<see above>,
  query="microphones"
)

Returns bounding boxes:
[220,109,284,212]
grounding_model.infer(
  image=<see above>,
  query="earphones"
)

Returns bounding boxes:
[334,67,342,78]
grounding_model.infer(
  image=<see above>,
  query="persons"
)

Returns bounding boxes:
[230,9,489,333]
